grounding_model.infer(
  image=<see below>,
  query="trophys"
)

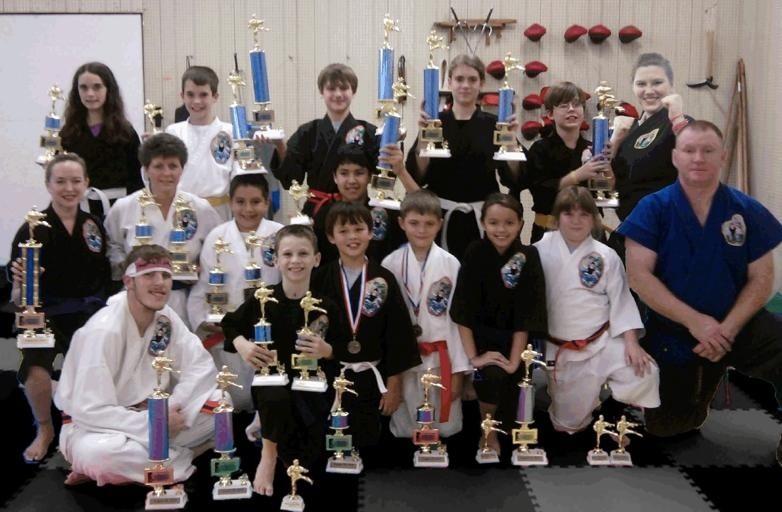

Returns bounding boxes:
[280,458,315,512]
[419,28,452,158]
[377,15,399,120]
[143,349,189,510]
[245,12,285,144]
